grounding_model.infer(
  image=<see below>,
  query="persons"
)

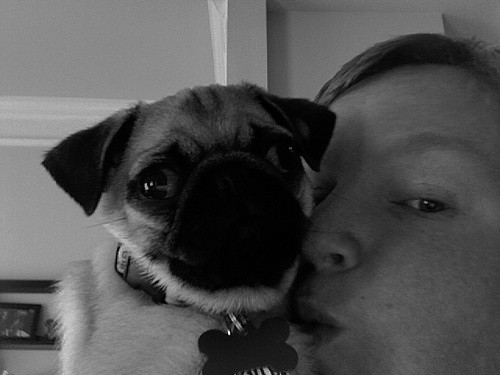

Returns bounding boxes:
[282,29,500,375]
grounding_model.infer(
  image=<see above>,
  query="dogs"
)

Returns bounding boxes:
[40,82,336,375]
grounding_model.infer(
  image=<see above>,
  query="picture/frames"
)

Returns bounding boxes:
[0,300,43,343]
